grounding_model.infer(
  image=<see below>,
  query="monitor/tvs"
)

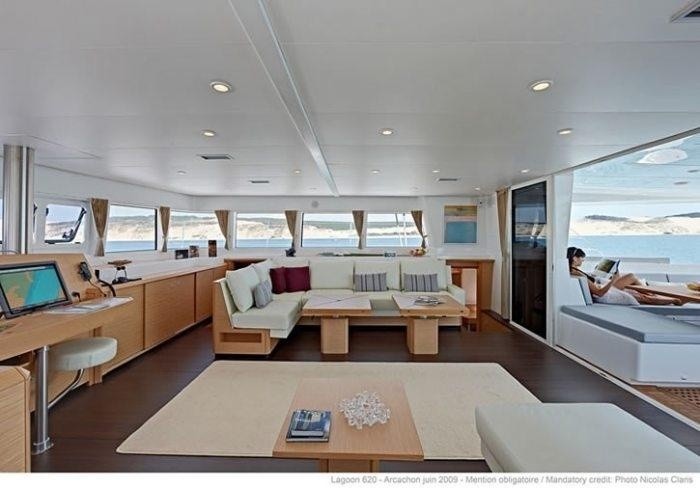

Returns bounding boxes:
[0,261,73,319]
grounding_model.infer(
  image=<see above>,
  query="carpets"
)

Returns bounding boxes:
[114,360,545,460]
[632,386,700,426]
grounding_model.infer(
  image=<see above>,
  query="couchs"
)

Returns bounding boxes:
[211,278,467,359]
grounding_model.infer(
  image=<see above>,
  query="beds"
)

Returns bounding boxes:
[556,302,700,389]
[556,274,700,326]
[474,402,700,472]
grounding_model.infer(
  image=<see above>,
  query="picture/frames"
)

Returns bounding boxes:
[440,203,478,247]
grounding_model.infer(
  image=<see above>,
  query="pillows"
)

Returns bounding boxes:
[225,255,447,312]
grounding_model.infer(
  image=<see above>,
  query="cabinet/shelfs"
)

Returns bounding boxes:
[445,258,495,311]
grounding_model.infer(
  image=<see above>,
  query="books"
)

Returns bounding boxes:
[413,294,447,306]
[45,300,109,316]
[589,257,620,286]
[285,412,333,442]
[290,409,325,436]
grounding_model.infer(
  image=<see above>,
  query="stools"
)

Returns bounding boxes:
[46,336,118,410]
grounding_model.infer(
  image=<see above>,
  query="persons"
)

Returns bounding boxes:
[567,246,690,306]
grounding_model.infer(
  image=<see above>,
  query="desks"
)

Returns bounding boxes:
[1,254,135,457]
